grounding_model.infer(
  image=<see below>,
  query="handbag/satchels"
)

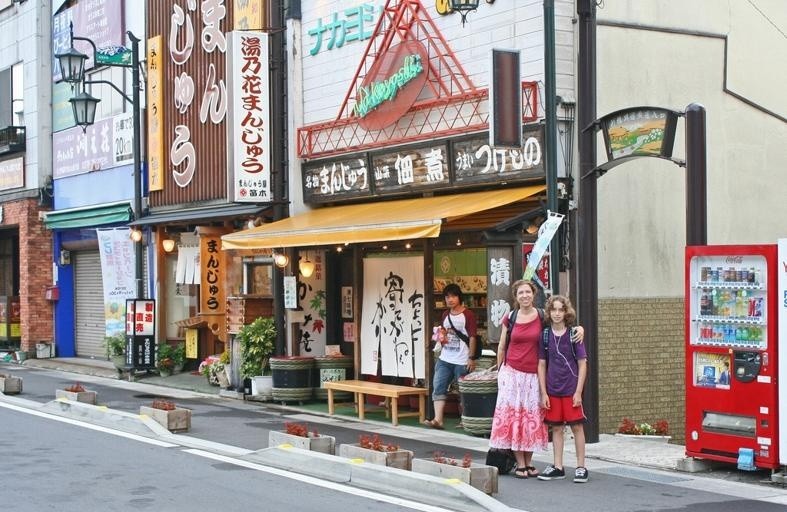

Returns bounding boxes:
[448,308,482,359]
[485,447,516,475]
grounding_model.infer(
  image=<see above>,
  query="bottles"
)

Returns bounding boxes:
[700,266,761,284]
[476,314,485,328]
[697,319,762,345]
[466,294,487,308]
[698,286,750,318]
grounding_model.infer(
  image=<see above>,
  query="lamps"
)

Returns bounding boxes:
[130,225,143,243]
[161,227,175,253]
[298,250,316,277]
[274,247,289,268]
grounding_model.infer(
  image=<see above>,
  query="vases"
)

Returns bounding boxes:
[614,432,670,444]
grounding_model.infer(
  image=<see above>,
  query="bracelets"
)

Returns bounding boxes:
[469,356,476,360]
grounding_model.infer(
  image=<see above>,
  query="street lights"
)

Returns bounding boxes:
[54,20,156,376]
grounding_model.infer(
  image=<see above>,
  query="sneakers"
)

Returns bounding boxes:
[537,465,566,480]
[573,467,589,483]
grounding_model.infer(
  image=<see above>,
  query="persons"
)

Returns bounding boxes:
[423,283,478,430]
[488,280,584,480]
[534,294,590,483]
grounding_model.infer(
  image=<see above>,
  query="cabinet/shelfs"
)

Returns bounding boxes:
[434,290,487,330]
[225,294,274,334]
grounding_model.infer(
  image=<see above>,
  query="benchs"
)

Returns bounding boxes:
[324,378,429,427]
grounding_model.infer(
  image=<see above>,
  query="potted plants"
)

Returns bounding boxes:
[100,332,125,369]
[0,373,23,394]
[56,383,96,406]
[139,400,192,431]
[339,432,412,469]
[412,450,498,496]
[269,420,336,456]
[239,315,272,397]
[156,343,187,376]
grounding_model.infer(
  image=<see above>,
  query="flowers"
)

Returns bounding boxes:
[615,418,671,435]
[198,348,230,386]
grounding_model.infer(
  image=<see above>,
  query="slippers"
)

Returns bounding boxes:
[515,467,528,478]
[423,419,445,430]
[526,467,539,477]
